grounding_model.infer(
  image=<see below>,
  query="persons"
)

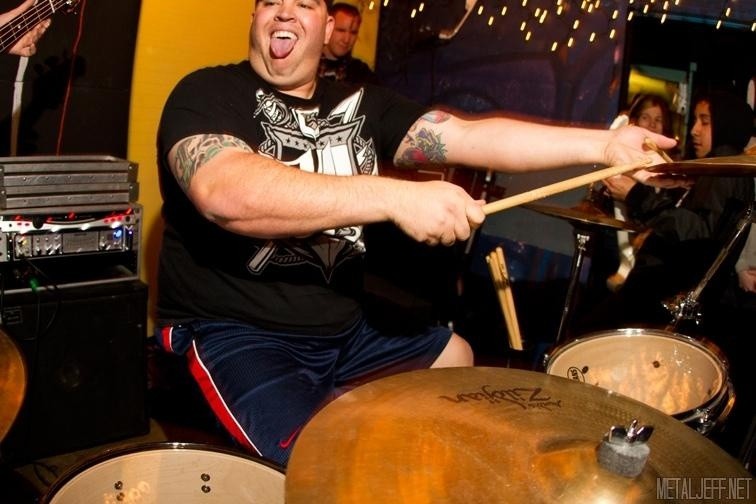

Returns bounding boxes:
[320,0,380,84]
[152,0,696,470]
[595,88,756,336]
[628,90,671,137]
[735,221,756,293]
[0,0,51,57]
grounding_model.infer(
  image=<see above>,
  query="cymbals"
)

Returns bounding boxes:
[520,201,644,232]
[646,155,756,173]
[0,330,29,442]
[285,366,756,504]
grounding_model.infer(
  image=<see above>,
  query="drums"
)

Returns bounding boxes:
[43,442,288,504]
[543,328,737,436]
